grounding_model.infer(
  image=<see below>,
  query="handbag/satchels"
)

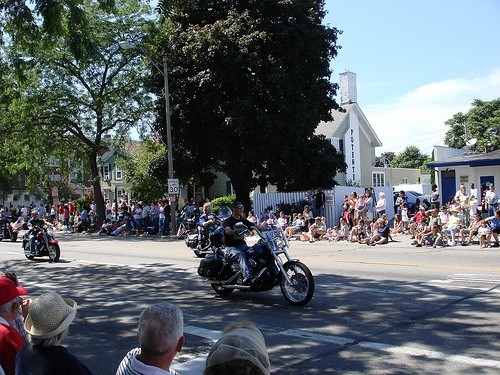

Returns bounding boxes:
[197,259,222,279]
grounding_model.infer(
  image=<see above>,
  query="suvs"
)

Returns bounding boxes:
[393,190,431,213]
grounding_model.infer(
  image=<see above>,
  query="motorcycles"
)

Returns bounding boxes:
[21,220,60,262]
[186,214,214,258]
[0,214,18,242]
[197,215,315,306]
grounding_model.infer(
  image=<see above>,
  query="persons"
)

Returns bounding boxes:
[115,303,185,375]
[0,200,171,256]
[202,319,270,375]
[246,187,397,246]
[390,182,500,249]
[176,197,271,284]
[0,270,97,375]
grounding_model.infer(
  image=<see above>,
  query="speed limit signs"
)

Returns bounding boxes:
[168,179,179,195]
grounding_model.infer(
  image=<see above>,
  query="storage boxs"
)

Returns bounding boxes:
[208,232,223,248]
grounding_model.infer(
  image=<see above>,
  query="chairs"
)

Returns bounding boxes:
[289,216,306,239]
[441,221,480,245]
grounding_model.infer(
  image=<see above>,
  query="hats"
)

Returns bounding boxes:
[451,207,460,212]
[23,292,77,339]
[230,201,242,209]
[418,206,425,211]
[0,277,29,304]
[314,217,322,220]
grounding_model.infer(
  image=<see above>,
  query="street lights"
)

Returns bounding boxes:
[118,41,177,236]
